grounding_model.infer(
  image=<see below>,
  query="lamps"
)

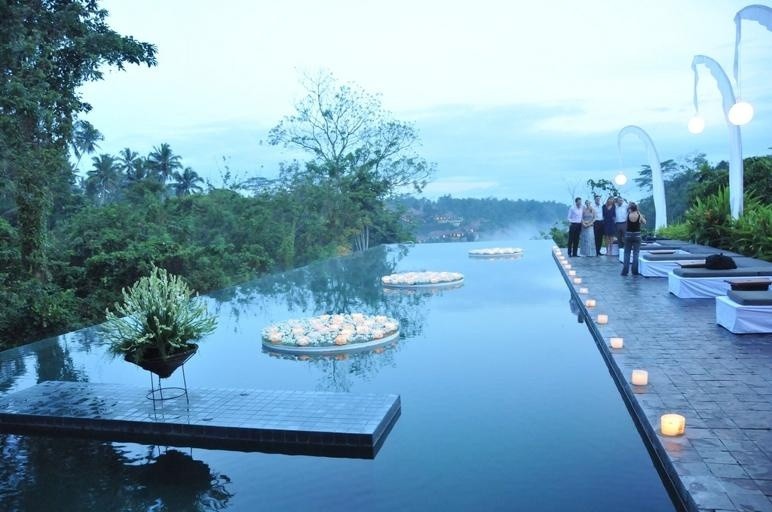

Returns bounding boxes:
[550,241,688,440]
[727,8,755,126]
[687,57,706,133]
[614,138,628,186]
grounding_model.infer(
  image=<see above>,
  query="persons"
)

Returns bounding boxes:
[621,203,647,276]
[567,197,583,257]
[614,197,628,248]
[580,200,596,257]
[602,197,616,255]
[593,196,604,256]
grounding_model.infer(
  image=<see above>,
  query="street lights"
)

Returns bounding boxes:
[685,55,743,232]
[613,125,667,239]
[726,4,772,126]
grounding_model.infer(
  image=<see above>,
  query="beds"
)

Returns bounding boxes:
[611,237,772,337]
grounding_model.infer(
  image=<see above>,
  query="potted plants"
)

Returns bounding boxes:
[98,259,222,401]
